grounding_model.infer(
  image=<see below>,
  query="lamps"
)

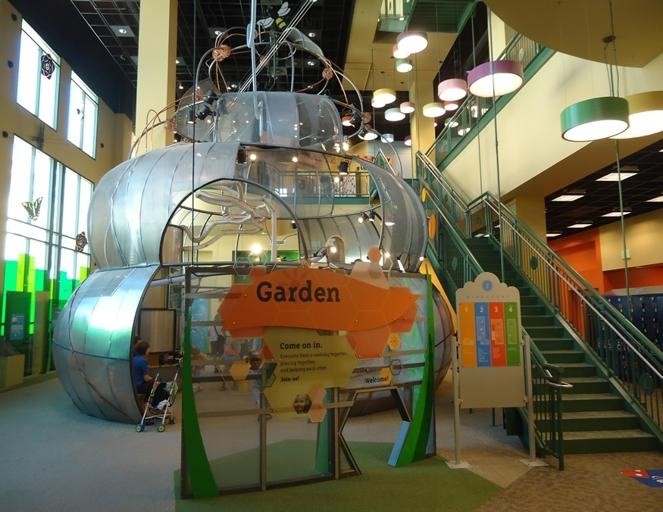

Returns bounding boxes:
[422,6,524,136]
[560,36,662,142]
[393,1,429,74]
[342,72,415,147]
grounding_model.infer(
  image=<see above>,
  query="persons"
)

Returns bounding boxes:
[131,342,153,395]
[158,350,176,365]
[221,343,239,361]
[189,344,207,361]
[292,393,306,413]
[131,335,142,351]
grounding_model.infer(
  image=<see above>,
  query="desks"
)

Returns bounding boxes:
[147,363,182,370]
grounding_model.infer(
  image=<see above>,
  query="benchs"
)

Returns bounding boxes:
[135,392,145,414]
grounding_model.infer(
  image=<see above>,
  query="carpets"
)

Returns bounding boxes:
[172,438,504,511]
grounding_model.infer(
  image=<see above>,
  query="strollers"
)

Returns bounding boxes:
[136,365,180,433]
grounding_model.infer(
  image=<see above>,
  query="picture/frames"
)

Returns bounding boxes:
[168,284,184,310]
[168,266,181,285]
